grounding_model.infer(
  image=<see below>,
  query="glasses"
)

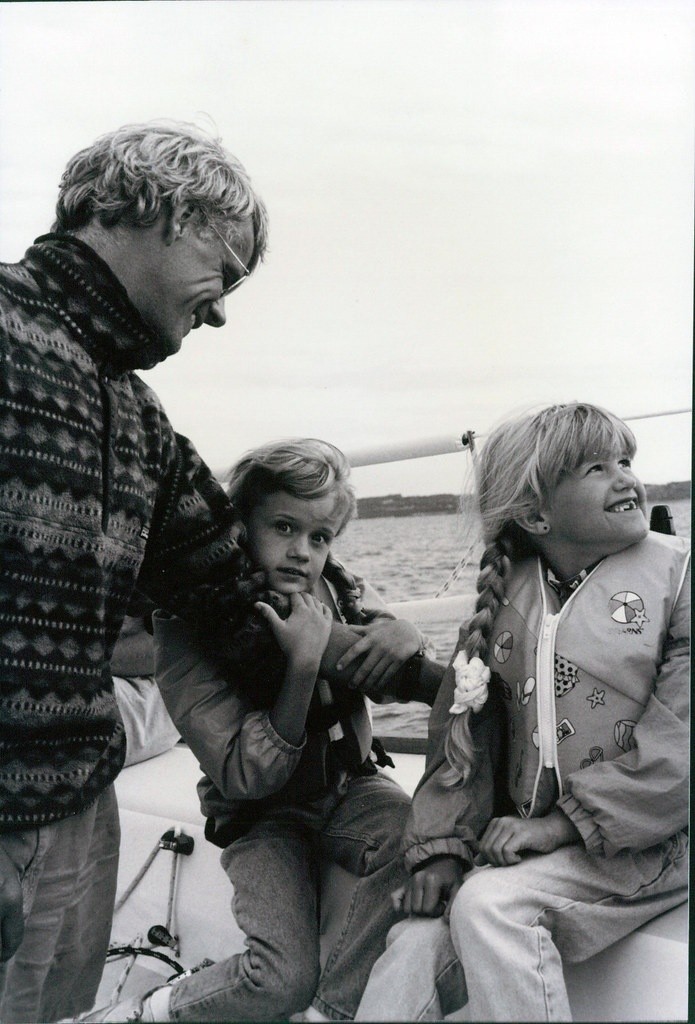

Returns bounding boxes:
[200,208,252,296]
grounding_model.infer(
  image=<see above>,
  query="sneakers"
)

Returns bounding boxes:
[74,982,167,1023]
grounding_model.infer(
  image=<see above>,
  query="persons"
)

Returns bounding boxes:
[353,402,690,1022]
[0,117,448,1024]
[79,439,437,1024]
